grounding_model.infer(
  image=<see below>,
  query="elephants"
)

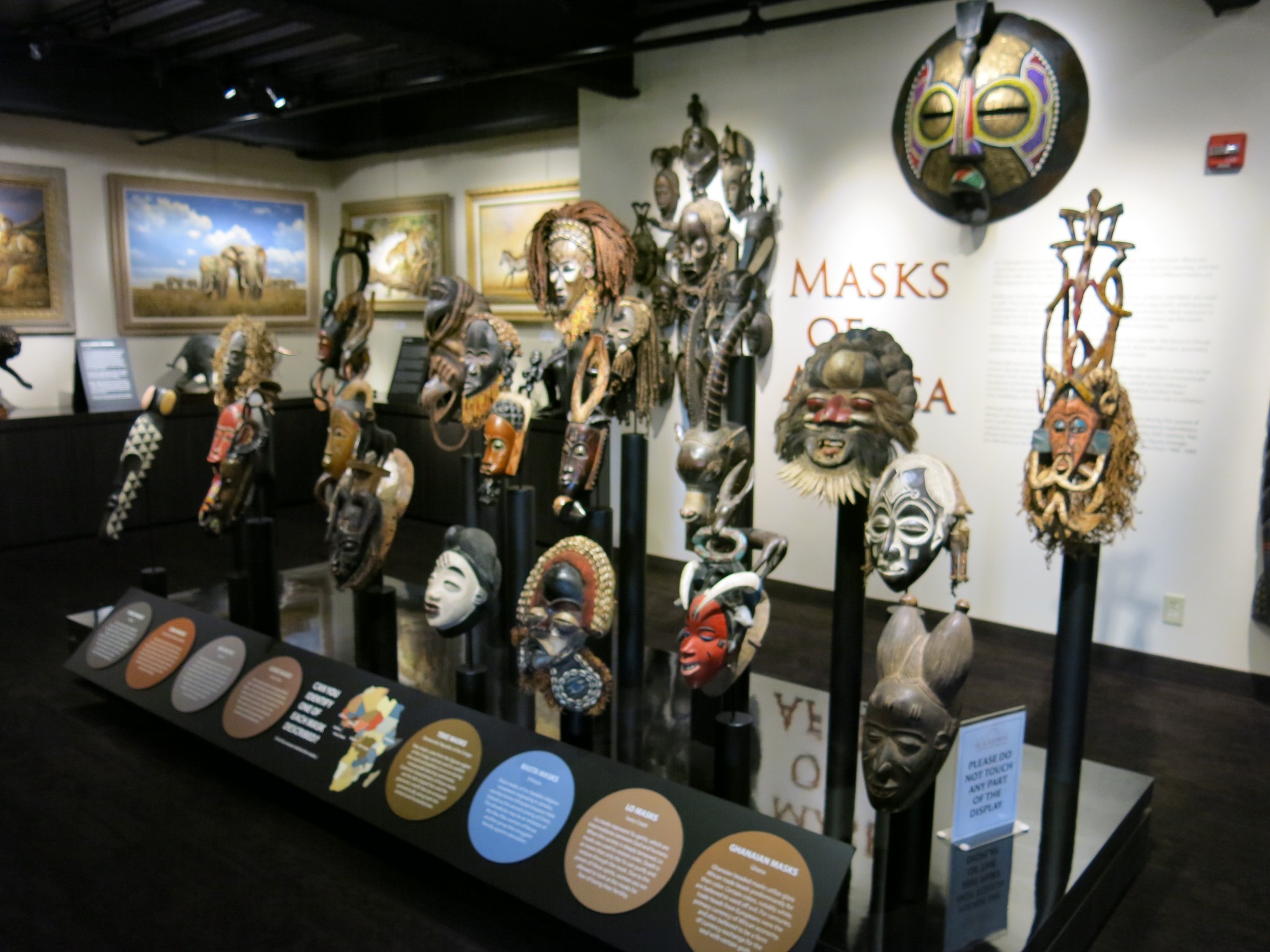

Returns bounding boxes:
[150,241,269,303]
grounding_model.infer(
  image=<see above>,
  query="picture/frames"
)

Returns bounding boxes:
[107,170,320,337]
[340,194,454,313]
[464,180,579,323]
[0,161,77,335]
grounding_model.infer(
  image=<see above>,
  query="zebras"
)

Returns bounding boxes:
[496,248,532,290]
